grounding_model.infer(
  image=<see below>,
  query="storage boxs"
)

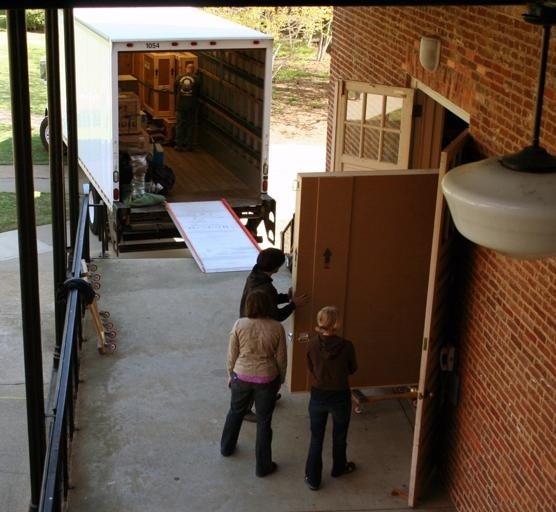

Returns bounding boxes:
[118,75,139,95]
[118,91,141,135]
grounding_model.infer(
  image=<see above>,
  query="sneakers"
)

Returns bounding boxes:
[243,411,258,422]
[304,474,319,490]
[332,461,356,478]
[256,461,276,477]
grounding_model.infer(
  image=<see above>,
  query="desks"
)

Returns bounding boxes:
[142,108,177,145]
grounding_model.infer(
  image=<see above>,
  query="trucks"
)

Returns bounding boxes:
[39,5,278,258]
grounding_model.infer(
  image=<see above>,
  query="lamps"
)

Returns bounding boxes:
[419,36,441,73]
[442,13,556,261]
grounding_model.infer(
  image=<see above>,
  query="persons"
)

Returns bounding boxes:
[304,305,357,491]
[238,247,311,422]
[220,289,278,479]
